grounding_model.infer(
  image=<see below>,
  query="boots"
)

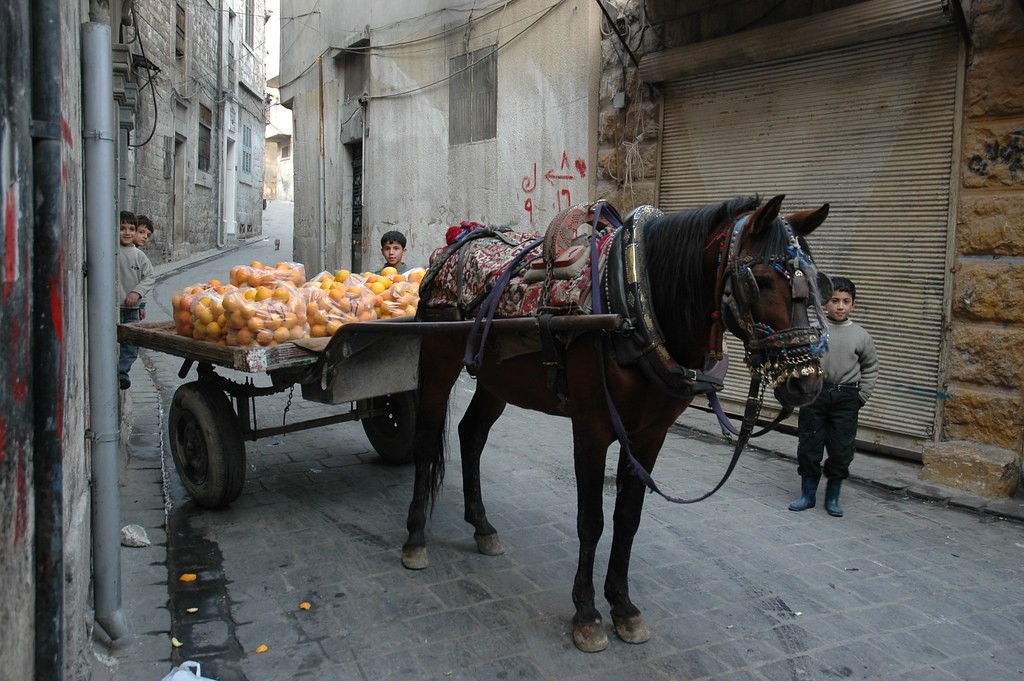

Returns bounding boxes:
[824,480,844,516]
[788,475,820,511]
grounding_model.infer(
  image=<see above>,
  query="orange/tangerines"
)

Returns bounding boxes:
[170,261,430,347]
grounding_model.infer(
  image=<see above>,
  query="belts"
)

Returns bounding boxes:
[822,382,861,391]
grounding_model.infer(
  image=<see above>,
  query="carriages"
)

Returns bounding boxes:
[115,191,833,655]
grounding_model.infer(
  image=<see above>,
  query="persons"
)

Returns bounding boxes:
[374,231,409,275]
[119,210,154,389]
[133,215,154,320]
[789,278,879,515]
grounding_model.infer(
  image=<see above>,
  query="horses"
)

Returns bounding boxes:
[401,190,830,653]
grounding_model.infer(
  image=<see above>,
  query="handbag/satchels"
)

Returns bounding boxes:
[170,260,429,350]
